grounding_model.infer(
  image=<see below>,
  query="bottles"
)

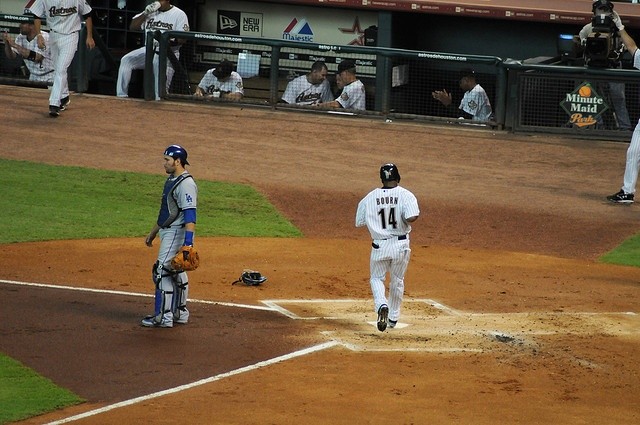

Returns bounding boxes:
[212,60,233,78]
[455,68,475,81]
[335,60,356,74]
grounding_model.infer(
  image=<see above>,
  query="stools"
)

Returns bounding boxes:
[160,224,185,229]
[366,236,406,249]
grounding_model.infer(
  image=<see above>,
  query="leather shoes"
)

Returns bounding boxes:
[144,3,155,16]
[612,10,625,31]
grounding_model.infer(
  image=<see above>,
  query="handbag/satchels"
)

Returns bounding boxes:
[185,231,193,245]
[24,50,36,61]
[37,33,42,36]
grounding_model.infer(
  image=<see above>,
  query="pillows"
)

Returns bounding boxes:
[380,164,399,183]
[164,144,191,166]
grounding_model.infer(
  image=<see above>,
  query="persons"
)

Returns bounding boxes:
[193,58,245,103]
[2,8,54,83]
[29,0,96,118]
[579,0,632,130]
[116,0,190,100]
[606,10,640,205]
[431,66,492,122]
[281,60,334,105]
[141,144,200,328]
[355,163,420,331]
[334,71,346,99]
[305,59,366,111]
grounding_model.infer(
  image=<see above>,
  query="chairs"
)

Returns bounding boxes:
[388,319,397,327]
[60,97,71,111]
[607,189,635,203]
[173,315,189,323]
[48,105,60,117]
[141,315,173,327]
[377,304,389,331]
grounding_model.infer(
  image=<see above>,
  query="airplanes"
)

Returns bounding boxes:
[242,272,266,286]
[171,245,198,273]
